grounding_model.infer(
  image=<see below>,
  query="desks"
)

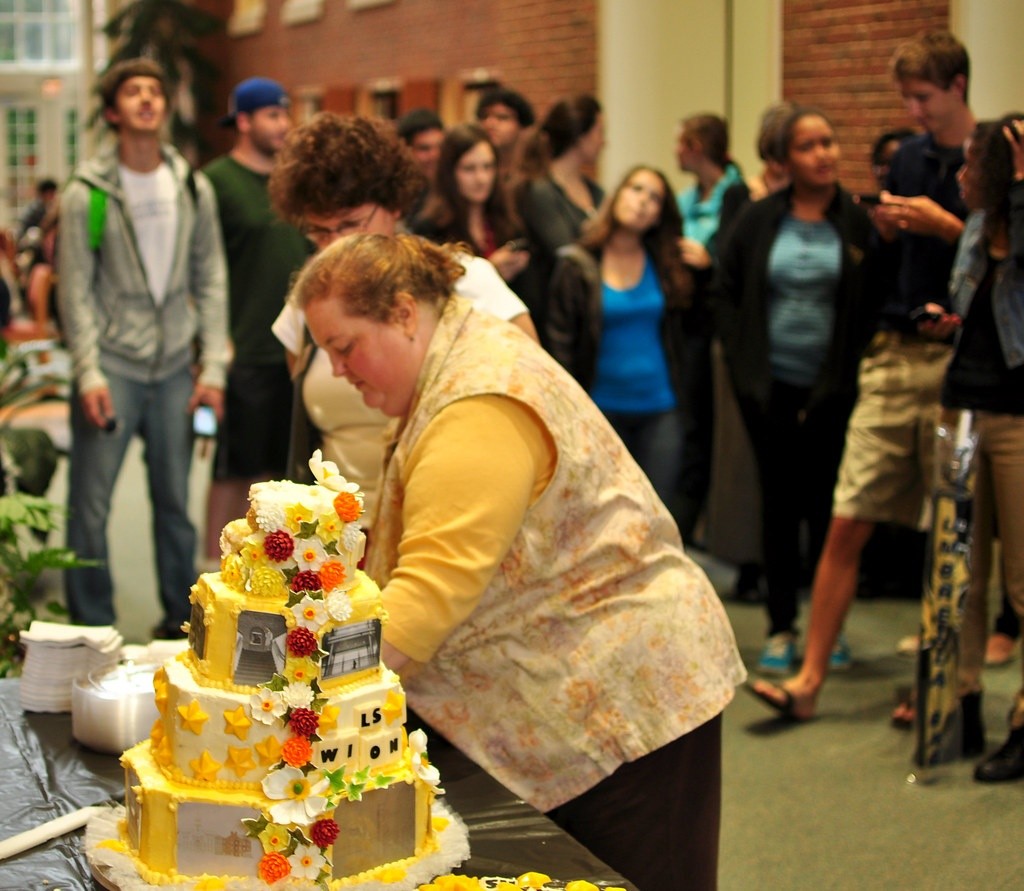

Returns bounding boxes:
[0,674,640,891]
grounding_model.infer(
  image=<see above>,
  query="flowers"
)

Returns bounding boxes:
[215,449,448,891]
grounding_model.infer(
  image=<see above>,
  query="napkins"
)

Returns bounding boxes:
[19,620,125,653]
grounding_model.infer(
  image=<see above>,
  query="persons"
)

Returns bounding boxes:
[405,125,531,301]
[395,109,446,180]
[913,114,1024,782]
[270,113,543,570]
[197,75,319,560]
[675,115,745,246]
[56,59,235,640]
[289,231,749,891]
[714,104,887,676]
[546,163,722,547]
[16,179,61,332]
[742,31,982,728]
[476,91,534,149]
[504,91,607,348]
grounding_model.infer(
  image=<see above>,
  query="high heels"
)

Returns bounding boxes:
[984,631,1024,668]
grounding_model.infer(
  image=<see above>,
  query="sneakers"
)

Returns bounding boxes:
[826,639,860,674]
[752,632,796,677]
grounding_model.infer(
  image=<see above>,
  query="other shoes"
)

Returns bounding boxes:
[917,693,987,763]
[973,727,1024,783]
[895,636,931,663]
[150,618,191,641]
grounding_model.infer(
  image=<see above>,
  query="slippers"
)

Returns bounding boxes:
[888,694,917,728]
[739,674,809,725]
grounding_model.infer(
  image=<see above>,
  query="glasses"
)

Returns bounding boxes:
[297,201,379,242]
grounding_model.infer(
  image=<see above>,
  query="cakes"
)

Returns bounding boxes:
[120,447,448,891]
[415,871,629,891]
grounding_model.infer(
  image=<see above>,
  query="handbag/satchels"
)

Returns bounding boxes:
[932,424,981,503]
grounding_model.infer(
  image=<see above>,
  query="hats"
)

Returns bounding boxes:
[213,76,290,128]
